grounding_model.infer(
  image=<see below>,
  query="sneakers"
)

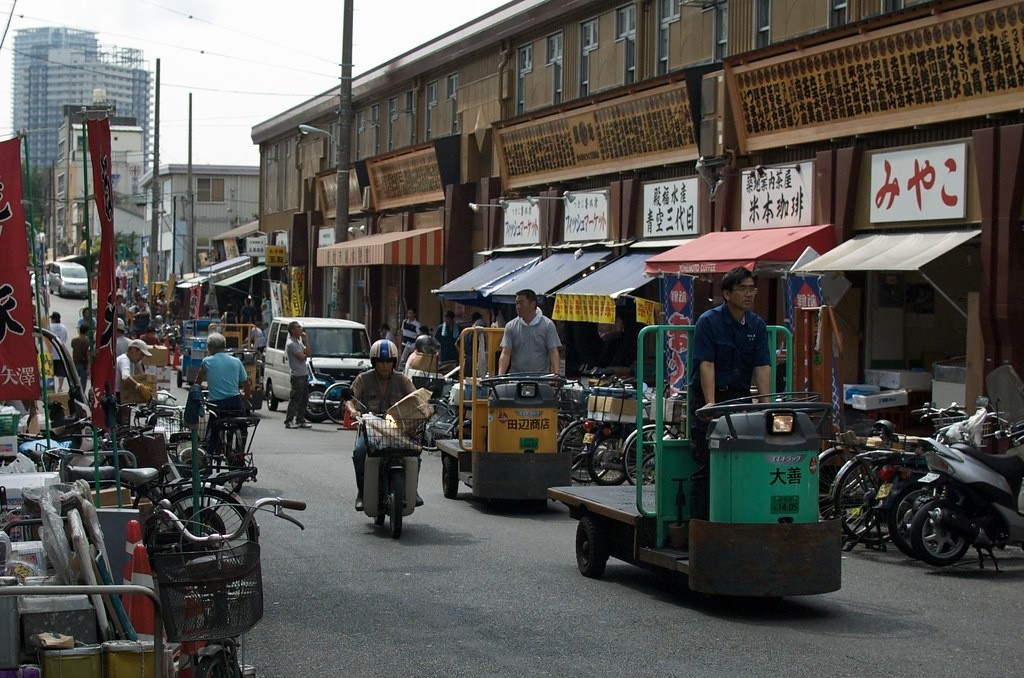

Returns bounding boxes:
[284,421,298,429]
[295,422,313,428]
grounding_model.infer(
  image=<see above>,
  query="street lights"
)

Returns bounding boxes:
[299,121,351,319]
[137,202,159,289]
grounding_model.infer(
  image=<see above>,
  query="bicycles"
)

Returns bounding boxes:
[816,404,1004,549]
[1,388,306,678]
[325,358,686,486]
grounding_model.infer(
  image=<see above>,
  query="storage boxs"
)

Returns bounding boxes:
[0,435,18,456]
[865,368,931,391]
[853,391,912,409]
[932,353,985,410]
[588,395,638,424]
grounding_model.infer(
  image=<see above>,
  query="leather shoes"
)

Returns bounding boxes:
[355,492,364,512]
[415,493,424,507]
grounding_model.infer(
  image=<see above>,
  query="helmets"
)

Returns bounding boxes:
[415,335,438,354]
[369,339,399,370]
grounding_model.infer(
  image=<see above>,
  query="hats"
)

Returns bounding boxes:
[127,339,153,358]
[117,317,127,330]
[49,312,62,323]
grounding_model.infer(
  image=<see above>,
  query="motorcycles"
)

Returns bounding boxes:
[342,387,429,539]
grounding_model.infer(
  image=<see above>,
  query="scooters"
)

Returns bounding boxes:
[870,421,1024,569]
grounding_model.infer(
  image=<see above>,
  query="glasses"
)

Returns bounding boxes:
[733,287,758,294]
[375,357,395,364]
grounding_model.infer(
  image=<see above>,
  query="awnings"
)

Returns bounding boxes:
[431,248,674,305]
[792,227,982,321]
[213,263,269,298]
[644,223,836,281]
[175,256,253,288]
[316,227,443,267]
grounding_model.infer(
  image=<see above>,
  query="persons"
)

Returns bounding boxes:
[374,308,491,407]
[284,321,313,429]
[49,288,186,428]
[498,288,561,393]
[688,267,773,523]
[194,332,252,460]
[344,340,426,511]
[218,297,271,353]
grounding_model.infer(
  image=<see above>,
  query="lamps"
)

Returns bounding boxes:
[498,200,537,209]
[469,203,509,212]
[527,196,567,207]
[298,125,335,141]
[755,164,801,178]
[711,172,756,183]
[563,190,609,204]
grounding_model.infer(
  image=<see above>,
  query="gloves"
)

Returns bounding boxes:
[138,384,153,400]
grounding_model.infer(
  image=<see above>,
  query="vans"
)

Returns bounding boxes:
[263,316,376,411]
[48,263,87,297]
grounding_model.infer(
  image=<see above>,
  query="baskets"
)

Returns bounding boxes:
[933,412,992,449]
[146,407,211,445]
[151,540,264,643]
[360,417,428,458]
